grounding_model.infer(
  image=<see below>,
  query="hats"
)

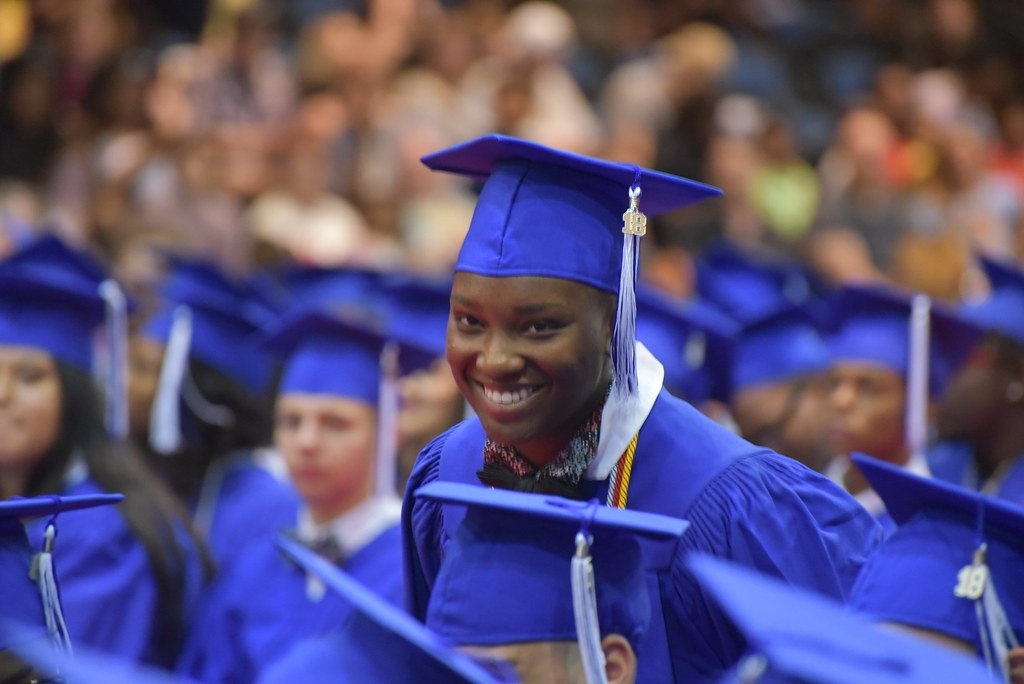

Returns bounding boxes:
[418,132,725,398]
[0,234,1024,498]
[415,476,692,649]
[850,451,1023,683]
[0,489,154,659]
[678,545,1011,683]
[250,534,497,684]
[0,615,194,684]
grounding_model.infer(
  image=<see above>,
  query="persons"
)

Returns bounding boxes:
[402,134,878,624]
[0,0,1024,684]
